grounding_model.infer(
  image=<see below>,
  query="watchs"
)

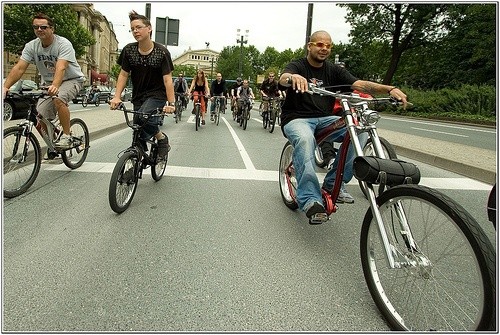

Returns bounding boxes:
[168,102,175,106]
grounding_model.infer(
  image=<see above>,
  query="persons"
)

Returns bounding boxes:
[237,79,255,123]
[210,73,227,121]
[188,69,209,125]
[91,85,101,102]
[260,72,283,124]
[111,9,175,181]
[231,78,243,121]
[4,14,87,161]
[279,30,407,225]
[173,74,189,118]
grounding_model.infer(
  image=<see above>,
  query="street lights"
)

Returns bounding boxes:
[236,29,250,78]
[211,55,215,83]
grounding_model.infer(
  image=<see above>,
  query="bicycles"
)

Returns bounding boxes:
[314,100,397,200]
[82,85,100,108]
[211,96,226,126]
[278,78,495,331]
[173,91,188,124]
[189,93,210,131]
[261,96,285,133]
[230,96,255,130]
[222,97,228,114]
[3,85,91,199]
[107,99,173,214]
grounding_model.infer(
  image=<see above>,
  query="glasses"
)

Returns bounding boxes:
[316,41,331,49]
[33,25,53,29]
[130,26,147,32]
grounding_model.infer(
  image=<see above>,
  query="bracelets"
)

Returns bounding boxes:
[387,87,397,93]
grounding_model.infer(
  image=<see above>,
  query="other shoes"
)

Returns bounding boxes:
[338,189,355,204]
[156,140,172,160]
[306,201,326,219]
[57,135,75,149]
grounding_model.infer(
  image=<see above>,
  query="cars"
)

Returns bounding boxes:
[3,78,39,122]
[72,84,111,104]
[109,87,133,102]
